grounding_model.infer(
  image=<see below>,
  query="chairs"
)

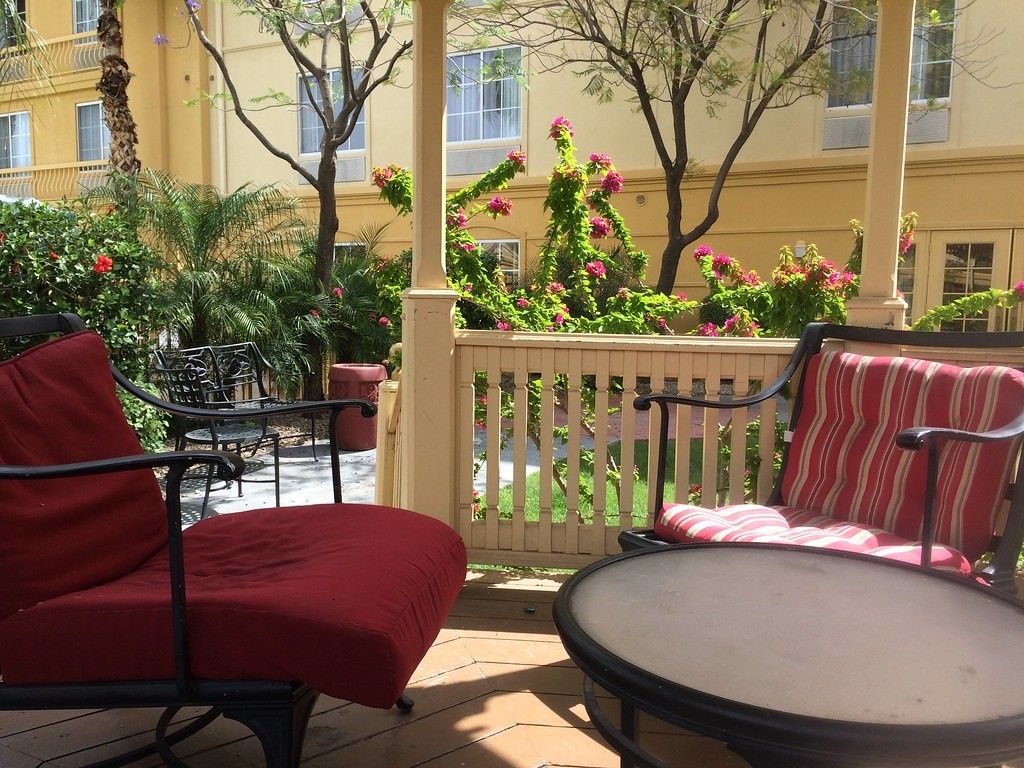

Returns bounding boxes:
[619,323,1024,599]
[152,347,281,520]
[0,313,472,768]
[183,342,317,487]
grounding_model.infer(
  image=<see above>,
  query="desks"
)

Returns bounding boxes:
[552,540,1024,768]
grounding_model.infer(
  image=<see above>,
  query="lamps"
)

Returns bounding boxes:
[795,240,806,257]
